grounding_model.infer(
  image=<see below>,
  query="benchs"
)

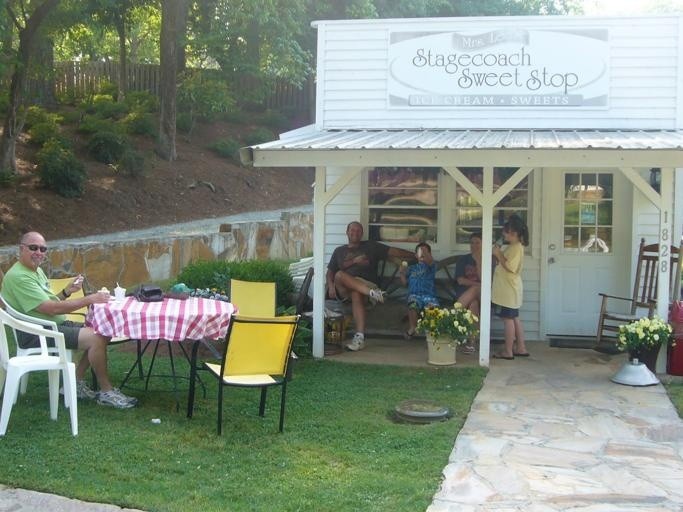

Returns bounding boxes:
[315,244,493,330]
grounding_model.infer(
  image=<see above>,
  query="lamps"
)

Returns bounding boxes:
[649,169,660,193]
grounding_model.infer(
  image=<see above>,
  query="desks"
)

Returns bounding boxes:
[87,297,234,416]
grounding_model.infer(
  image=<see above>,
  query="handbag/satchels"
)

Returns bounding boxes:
[135,284,164,302]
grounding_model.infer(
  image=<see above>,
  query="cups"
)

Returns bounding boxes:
[97,289,108,295]
[114,288,126,303]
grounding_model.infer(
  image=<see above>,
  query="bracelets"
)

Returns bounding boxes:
[399,272,404,275]
[61,289,71,298]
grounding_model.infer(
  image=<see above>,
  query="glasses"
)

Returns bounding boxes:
[20,242,46,252]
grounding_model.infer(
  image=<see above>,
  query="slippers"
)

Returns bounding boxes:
[493,348,514,359]
[463,344,475,354]
[403,331,414,339]
[513,350,530,356]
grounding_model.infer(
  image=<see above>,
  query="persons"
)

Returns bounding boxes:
[452,231,496,353]
[398,241,464,341]
[0,231,138,410]
[325,221,417,351]
[491,214,531,361]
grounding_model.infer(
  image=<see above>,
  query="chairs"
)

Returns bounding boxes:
[203,315,302,437]
[229,278,279,320]
[592,236,682,352]
[0,294,72,410]
[0,296,80,435]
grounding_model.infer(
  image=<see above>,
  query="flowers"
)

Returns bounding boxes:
[411,301,479,346]
[613,318,678,352]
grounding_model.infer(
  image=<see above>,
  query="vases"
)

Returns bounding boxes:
[628,343,661,372]
[424,330,457,366]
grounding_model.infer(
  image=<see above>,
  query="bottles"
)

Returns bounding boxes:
[495,237,503,249]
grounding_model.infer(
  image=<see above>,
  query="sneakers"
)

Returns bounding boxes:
[346,332,365,350]
[369,287,388,303]
[58,378,95,399]
[96,386,138,409]
